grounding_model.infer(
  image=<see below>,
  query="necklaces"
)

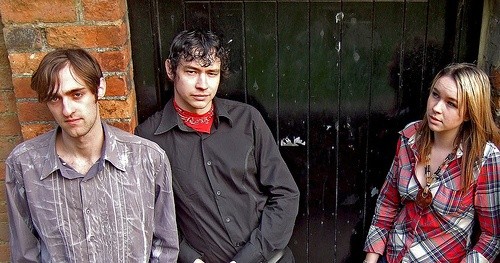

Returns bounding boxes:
[415,137,462,208]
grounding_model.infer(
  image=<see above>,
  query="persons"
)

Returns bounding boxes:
[132,27,301,263]
[4,46,181,263]
[362,64,500,263]
